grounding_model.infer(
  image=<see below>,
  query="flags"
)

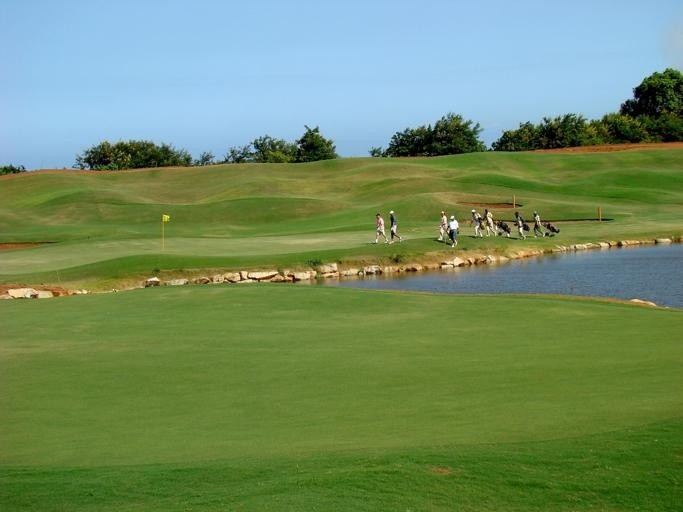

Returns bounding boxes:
[162,214,170,222]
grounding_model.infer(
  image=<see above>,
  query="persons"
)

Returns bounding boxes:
[371,213,388,244]
[389,210,402,245]
[448,215,459,247]
[470,209,560,239]
[437,210,452,241]
